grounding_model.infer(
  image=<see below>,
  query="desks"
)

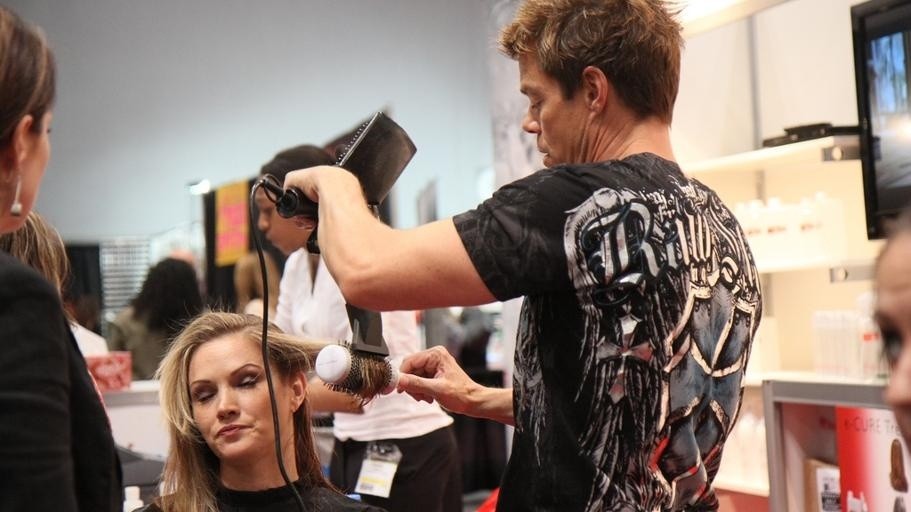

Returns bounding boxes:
[758,364,910,510]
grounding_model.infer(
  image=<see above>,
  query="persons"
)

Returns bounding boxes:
[893,496,906,512]
[889,440,908,492]
[108,258,210,380]
[1,5,124,512]
[872,209,911,454]
[255,146,466,511]
[285,2,763,512]
[130,310,383,511]
[1,209,67,307]
[65,311,107,356]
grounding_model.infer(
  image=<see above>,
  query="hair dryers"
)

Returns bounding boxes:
[247,173,391,358]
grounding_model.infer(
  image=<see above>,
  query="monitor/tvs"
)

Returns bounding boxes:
[850,0,911,240]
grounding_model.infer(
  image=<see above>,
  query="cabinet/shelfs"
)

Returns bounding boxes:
[671,125,894,502]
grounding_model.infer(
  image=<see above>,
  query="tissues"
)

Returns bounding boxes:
[70,322,133,395]
